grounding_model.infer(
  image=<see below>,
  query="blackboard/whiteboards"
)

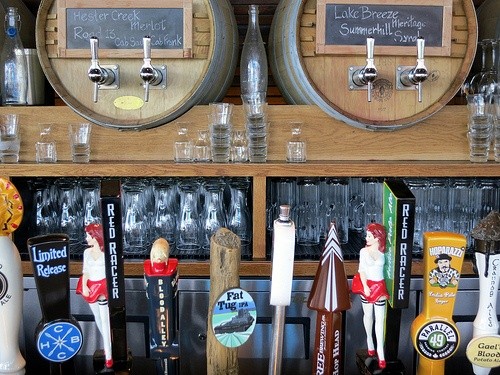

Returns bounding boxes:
[316,0,453,56]
[57,0,192,57]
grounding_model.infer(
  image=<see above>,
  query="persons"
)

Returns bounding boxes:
[352,223,391,369]
[76,223,114,368]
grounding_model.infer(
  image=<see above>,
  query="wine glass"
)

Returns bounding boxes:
[465,38,500,107]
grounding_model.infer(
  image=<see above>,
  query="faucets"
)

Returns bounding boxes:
[141,35,167,102]
[87,36,119,103]
[395,37,428,103]
[347,36,378,102]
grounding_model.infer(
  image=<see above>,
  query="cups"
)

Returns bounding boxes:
[465,93,500,163]
[209,102,233,163]
[0,114,20,163]
[119,178,249,253]
[267,178,500,259]
[241,92,268,163]
[68,122,92,163]
[28,177,104,256]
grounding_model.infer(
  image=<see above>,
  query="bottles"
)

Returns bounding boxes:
[0,6,28,104]
[35,123,57,165]
[12,48,44,106]
[240,4,268,100]
[173,126,210,162]
[285,121,307,164]
[230,129,248,162]
